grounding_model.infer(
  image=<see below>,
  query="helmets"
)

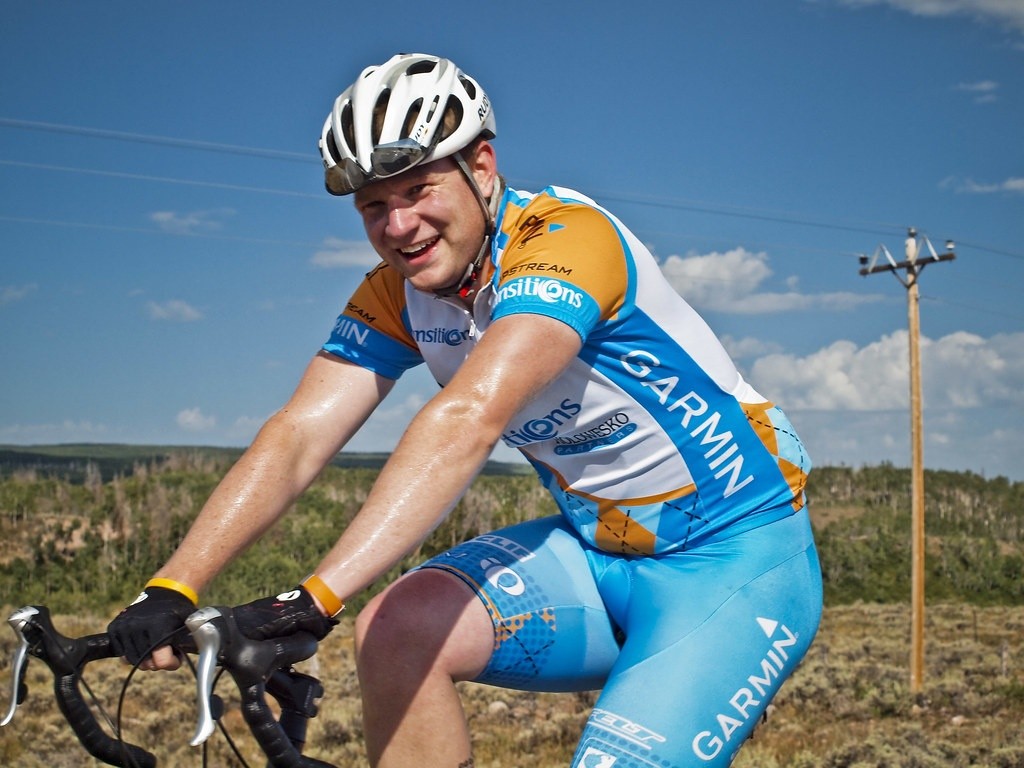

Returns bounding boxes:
[319,53,496,195]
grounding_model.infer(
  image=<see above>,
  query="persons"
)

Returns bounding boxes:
[103,53,823,767]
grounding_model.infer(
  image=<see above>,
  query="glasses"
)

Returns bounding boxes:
[324,112,445,195]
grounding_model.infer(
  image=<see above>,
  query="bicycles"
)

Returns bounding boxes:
[0,605,340,768]
